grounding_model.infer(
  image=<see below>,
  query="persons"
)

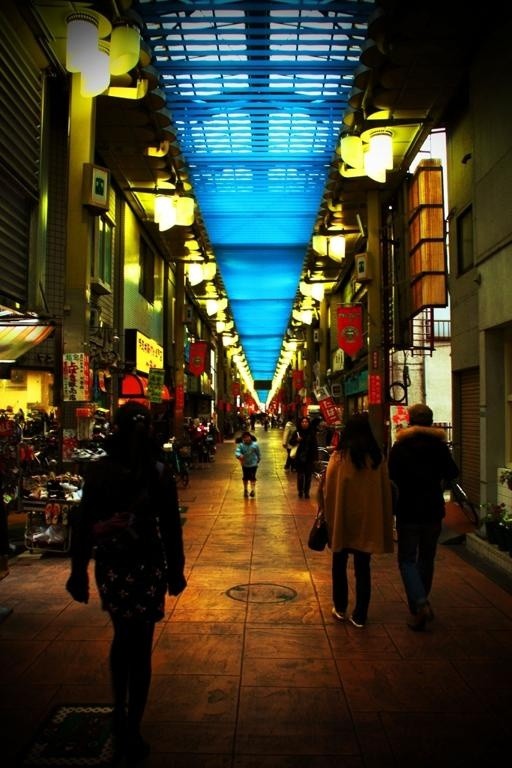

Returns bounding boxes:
[153,416,219,479]
[282,412,330,499]
[223,408,284,437]
[235,431,260,498]
[388,404,460,631]
[314,413,394,627]
[1,404,117,501]
[65,402,186,766]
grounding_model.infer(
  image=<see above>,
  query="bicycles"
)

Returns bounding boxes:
[170,440,190,488]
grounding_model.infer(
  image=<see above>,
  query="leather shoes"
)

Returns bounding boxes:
[416,607,435,625]
[409,624,433,632]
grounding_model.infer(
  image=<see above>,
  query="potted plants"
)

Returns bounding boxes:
[482,503,512,557]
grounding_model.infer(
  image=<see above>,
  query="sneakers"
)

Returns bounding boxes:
[244,489,248,497]
[332,607,345,622]
[348,615,365,628]
[250,491,254,497]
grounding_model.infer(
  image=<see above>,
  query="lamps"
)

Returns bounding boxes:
[153,173,239,345]
[63,1,146,103]
[337,126,407,187]
[291,219,359,331]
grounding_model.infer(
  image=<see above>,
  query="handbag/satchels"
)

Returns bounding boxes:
[307,511,327,551]
[441,482,482,534]
[289,431,300,460]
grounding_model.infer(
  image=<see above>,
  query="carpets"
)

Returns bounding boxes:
[14,702,129,767]
